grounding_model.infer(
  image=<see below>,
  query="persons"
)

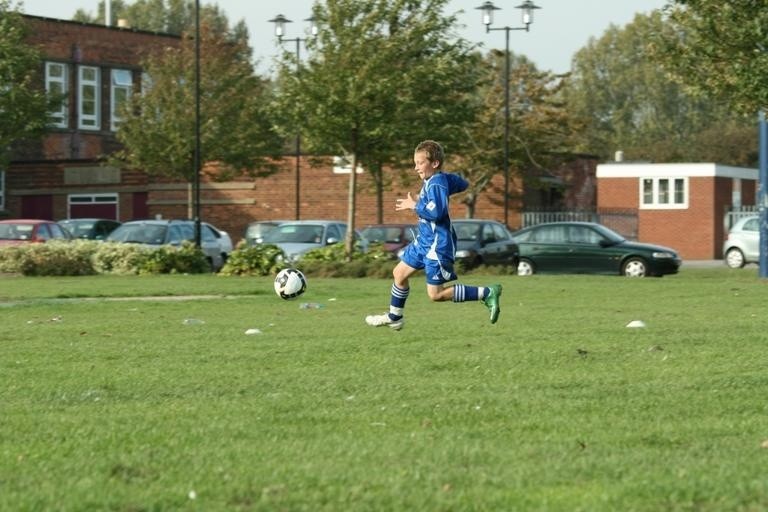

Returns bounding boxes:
[364,139,504,332]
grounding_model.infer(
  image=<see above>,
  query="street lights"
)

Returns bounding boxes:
[474,1,541,226]
[267,8,331,218]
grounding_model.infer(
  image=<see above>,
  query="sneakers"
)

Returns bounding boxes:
[365,311,404,331]
[480,284,502,324]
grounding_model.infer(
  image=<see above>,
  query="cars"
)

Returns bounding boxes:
[720,213,762,272]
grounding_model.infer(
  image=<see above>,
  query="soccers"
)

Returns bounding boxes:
[274,268,307,301]
